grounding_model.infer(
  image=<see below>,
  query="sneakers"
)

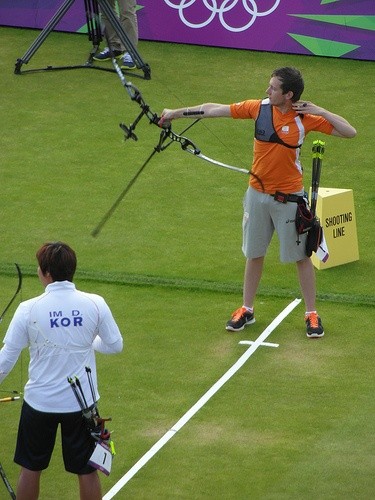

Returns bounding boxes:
[304,313,325,337]
[92,47,122,61]
[121,51,136,70]
[226,306,256,331]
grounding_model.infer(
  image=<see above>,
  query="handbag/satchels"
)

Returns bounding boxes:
[296,202,313,234]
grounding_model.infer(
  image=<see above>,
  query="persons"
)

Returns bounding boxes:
[93,0,139,69]
[158,67,357,338]
[0,241,123,500]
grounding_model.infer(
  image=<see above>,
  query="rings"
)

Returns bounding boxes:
[302,102,308,107]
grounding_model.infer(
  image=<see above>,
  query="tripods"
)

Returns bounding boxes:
[15,0,151,81]
